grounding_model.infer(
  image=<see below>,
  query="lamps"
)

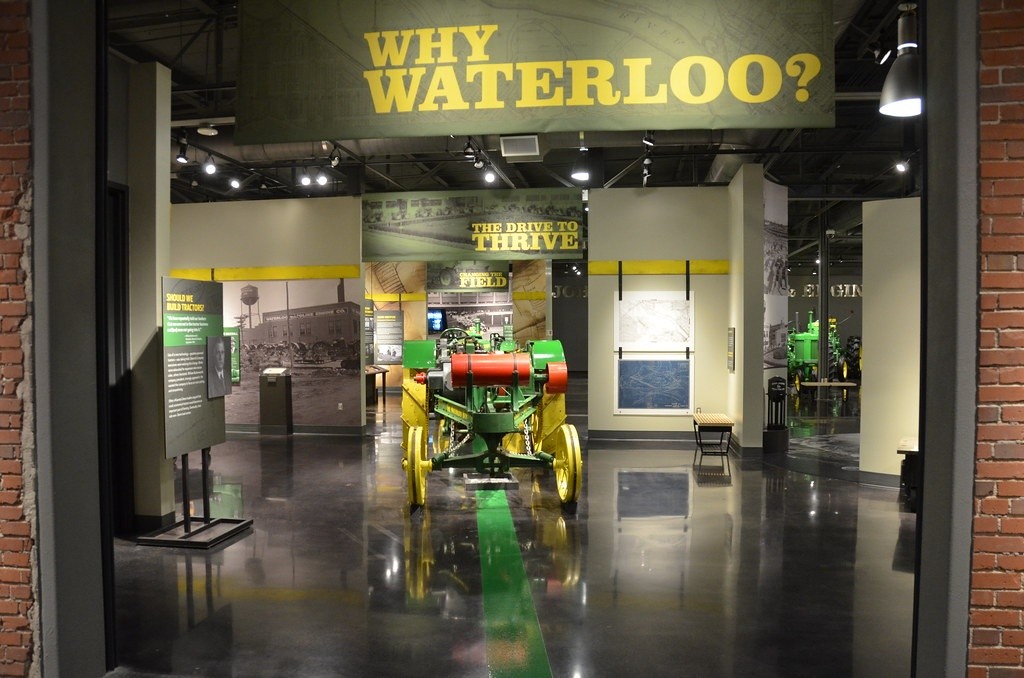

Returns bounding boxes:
[174,143,496,188]
[643,130,654,185]
[815,250,820,263]
[879,3,923,117]
[812,268,817,275]
[896,156,911,172]
[788,266,792,272]
[198,123,220,136]
[569,151,591,181]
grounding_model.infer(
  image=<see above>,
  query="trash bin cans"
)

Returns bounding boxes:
[258,367,295,437]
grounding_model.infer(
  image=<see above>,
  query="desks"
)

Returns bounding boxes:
[800,381,858,425]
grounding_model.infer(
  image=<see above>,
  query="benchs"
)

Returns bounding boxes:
[692,446,733,488]
[693,412,734,454]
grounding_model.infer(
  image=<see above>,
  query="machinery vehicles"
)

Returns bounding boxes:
[375,506,587,624]
[786,309,862,395]
[400,319,584,505]
